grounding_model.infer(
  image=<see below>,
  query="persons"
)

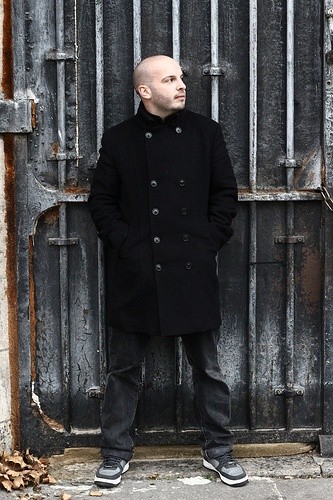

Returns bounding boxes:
[85,54,251,489]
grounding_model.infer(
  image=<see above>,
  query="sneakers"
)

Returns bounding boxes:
[202,450,249,487]
[94,456,130,487]
